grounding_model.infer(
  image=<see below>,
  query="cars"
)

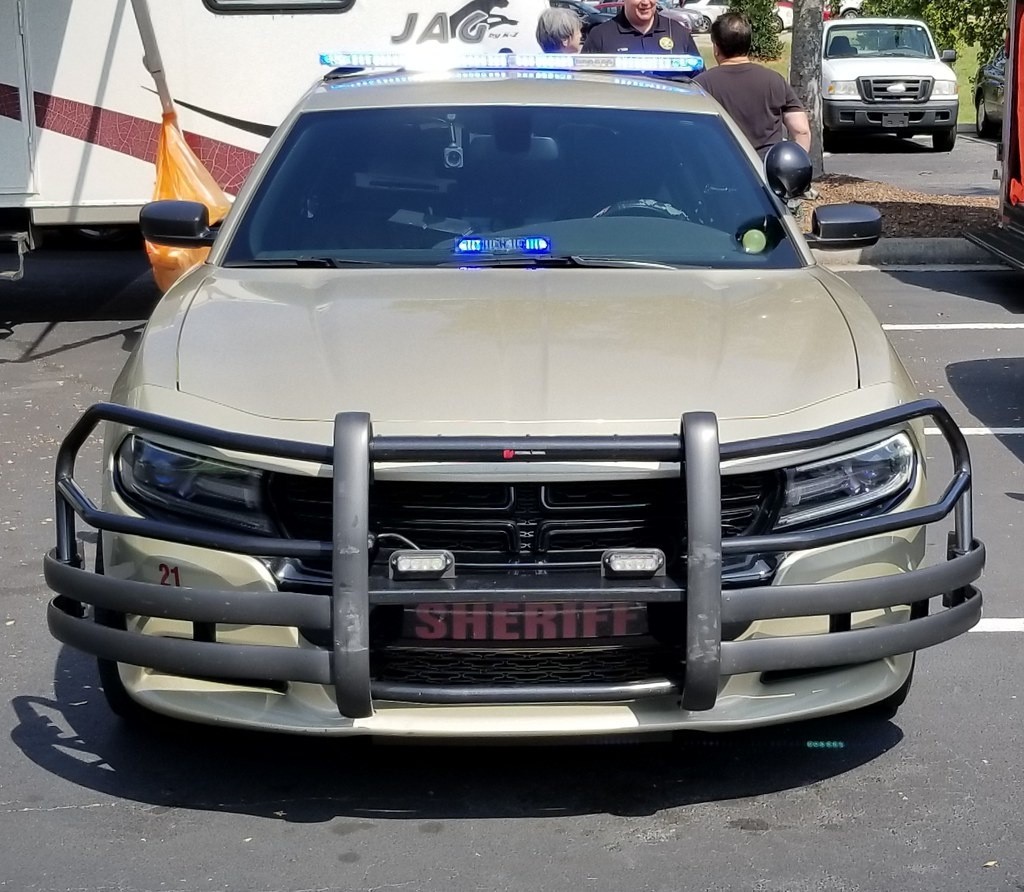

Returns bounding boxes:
[549,0,867,44]
[972,44,1008,138]
[42,52,985,736]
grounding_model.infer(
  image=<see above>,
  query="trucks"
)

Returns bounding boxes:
[0,0,554,280]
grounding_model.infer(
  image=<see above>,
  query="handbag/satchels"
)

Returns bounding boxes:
[144,113,232,293]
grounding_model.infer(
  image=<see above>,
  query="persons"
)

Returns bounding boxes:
[579,0,706,76]
[536,7,583,53]
[692,13,812,161]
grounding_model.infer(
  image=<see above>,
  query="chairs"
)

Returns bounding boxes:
[829,36,853,55]
[887,36,906,48]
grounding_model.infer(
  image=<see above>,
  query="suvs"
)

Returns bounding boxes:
[785,17,960,151]
